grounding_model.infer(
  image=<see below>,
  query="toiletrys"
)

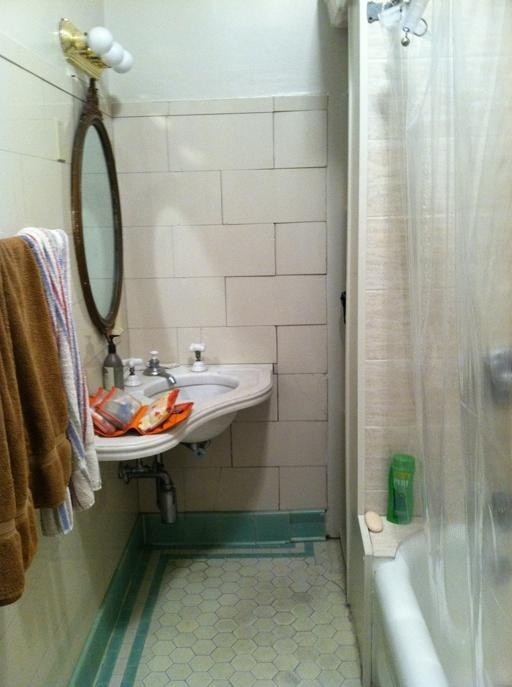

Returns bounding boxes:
[102,334,123,389]
[83,334,103,397]
[386,453,415,524]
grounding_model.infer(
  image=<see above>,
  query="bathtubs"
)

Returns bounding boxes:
[344,499,512,687]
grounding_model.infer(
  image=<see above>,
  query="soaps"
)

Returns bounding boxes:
[364,511,383,533]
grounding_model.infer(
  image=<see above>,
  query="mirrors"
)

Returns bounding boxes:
[70,79,128,329]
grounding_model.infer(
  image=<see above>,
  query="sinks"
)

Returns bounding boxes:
[93,363,273,462]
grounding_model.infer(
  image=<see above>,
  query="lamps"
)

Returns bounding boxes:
[57,14,136,81]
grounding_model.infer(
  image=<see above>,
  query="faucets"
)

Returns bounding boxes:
[141,350,177,386]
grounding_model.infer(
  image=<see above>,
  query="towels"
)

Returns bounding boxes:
[1,235,75,608]
[14,226,106,539]
